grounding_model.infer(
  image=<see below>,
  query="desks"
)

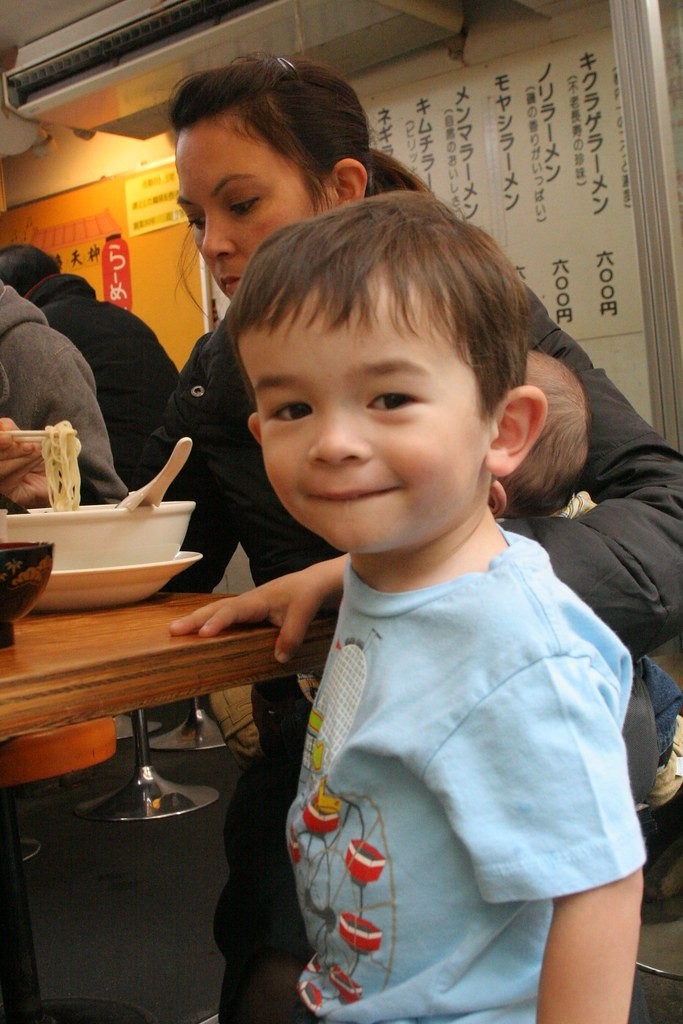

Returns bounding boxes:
[0,591,335,750]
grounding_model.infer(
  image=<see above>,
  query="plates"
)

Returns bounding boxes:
[35,550,203,611]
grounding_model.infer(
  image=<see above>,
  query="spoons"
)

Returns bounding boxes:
[114,437,193,510]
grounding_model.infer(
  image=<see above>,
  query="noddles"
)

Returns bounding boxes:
[40,421,82,512]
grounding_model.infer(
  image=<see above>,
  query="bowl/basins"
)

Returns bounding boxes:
[8,500,195,570]
[0,542,55,649]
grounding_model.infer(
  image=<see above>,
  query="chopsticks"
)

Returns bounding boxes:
[0,428,76,442]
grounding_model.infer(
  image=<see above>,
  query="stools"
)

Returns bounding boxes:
[74,708,216,822]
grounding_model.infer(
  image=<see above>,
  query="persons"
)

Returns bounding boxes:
[486,351,683,809]
[170,195,649,1024]
[0,59,682,1024]
[0,279,131,504]
[0,244,180,489]
